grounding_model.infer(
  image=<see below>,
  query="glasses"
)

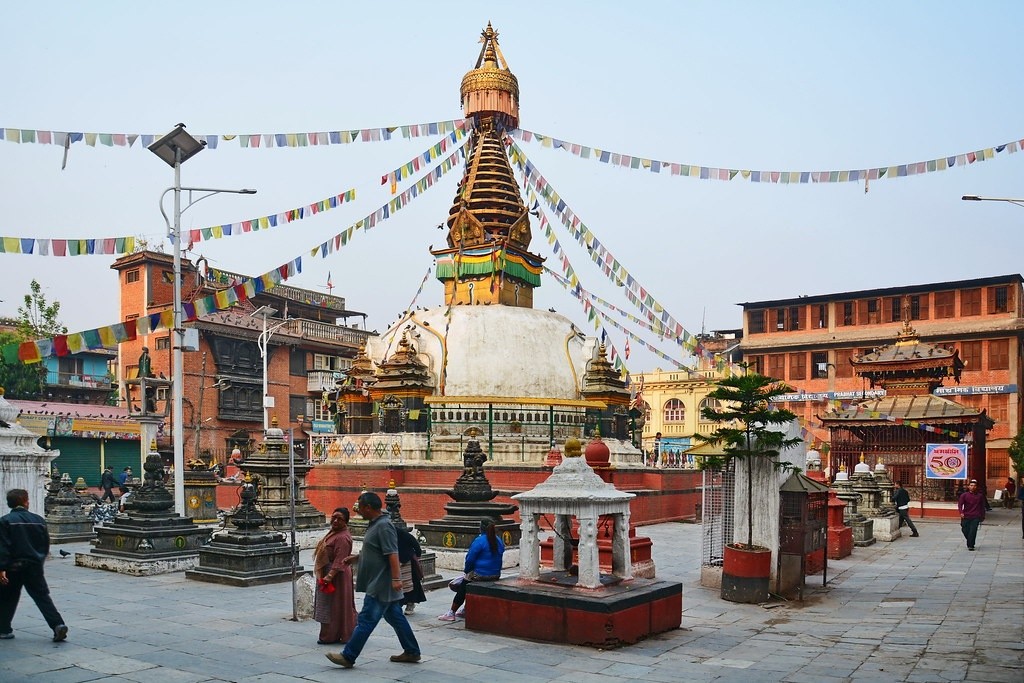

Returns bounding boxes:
[332,516,346,523]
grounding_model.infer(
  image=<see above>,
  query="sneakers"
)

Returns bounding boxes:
[455,609,465,617]
[438,612,455,621]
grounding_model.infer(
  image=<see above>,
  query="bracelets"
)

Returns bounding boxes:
[391,579,401,581]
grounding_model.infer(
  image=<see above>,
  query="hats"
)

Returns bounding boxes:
[970,480,978,483]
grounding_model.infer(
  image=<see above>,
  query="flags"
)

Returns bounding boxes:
[498,124,1024,455]
[0,114,479,369]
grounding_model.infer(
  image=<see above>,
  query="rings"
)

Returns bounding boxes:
[1,578,4,579]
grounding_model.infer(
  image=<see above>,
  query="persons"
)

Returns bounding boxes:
[325,492,421,668]
[396,527,427,614]
[438,518,505,621]
[312,508,359,644]
[99,465,133,512]
[0,489,68,642]
[957,478,986,551]
[891,481,920,537]
[987,476,1024,538]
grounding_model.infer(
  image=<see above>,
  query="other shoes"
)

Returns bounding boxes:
[325,652,355,668]
[968,546,975,551]
[317,640,326,644]
[390,652,421,662]
[910,532,919,537]
[0,631,15,639]
[53,624,68,642]
[405,608,414,614]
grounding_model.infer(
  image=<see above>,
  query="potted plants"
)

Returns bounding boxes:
[692,361,804,578]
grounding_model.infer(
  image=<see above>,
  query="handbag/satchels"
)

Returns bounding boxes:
[449,576,465,592]
[894,506,900,512]
[1008,493,1016,498]
[417,565,424,577]
